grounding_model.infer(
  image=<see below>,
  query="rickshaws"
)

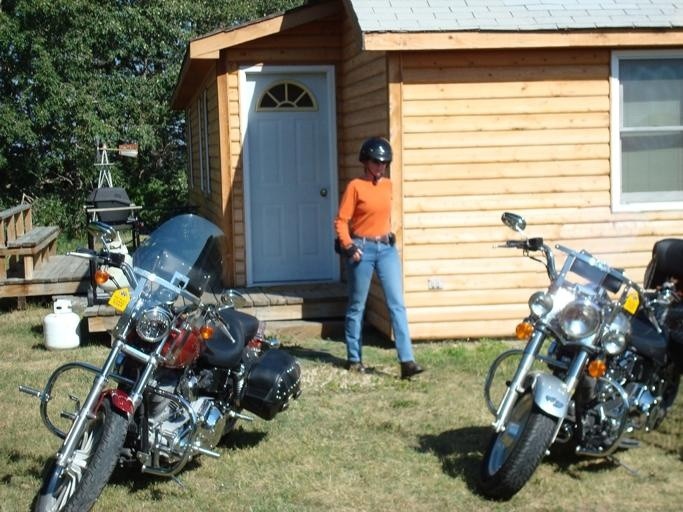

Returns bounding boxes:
[86,187,142,250]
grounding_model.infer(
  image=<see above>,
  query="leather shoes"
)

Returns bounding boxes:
[348,361,375,374]
[401,361,426,379]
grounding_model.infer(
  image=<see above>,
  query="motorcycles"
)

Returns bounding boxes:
[20,213,302,512]
[477,212,683,500]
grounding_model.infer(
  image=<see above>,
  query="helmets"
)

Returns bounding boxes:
[359,137,392,168]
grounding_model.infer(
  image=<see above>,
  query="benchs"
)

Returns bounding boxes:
[0,201,61,279]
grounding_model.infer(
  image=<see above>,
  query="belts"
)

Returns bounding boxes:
[350,233,389,244]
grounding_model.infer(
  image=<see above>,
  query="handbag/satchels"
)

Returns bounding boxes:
[334,238,344,255]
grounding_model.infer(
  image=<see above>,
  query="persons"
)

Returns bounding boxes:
[334,136,425,379]
